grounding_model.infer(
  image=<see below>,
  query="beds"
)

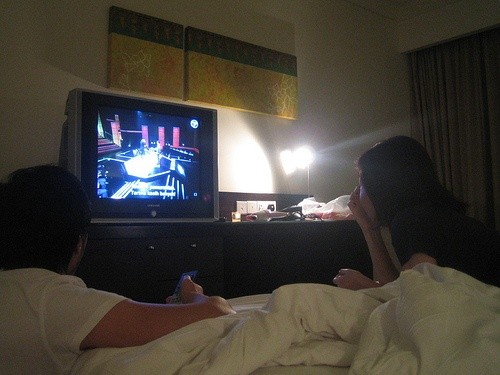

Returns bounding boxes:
[63,266,500,375]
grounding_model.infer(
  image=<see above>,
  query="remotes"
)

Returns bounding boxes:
[173,270,199,302]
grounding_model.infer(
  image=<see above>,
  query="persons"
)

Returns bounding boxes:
[139,139,146,158]
[0,165,236,375]
[155,140,161,162]
[333,135,499,290]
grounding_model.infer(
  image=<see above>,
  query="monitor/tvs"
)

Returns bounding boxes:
[65,88,219,224]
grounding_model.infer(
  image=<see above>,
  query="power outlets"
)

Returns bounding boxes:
[263,200,276,213]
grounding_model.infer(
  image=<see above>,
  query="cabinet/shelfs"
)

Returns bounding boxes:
[79,214,372,305]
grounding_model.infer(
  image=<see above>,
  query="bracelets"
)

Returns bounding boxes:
[375,281,380,286]
